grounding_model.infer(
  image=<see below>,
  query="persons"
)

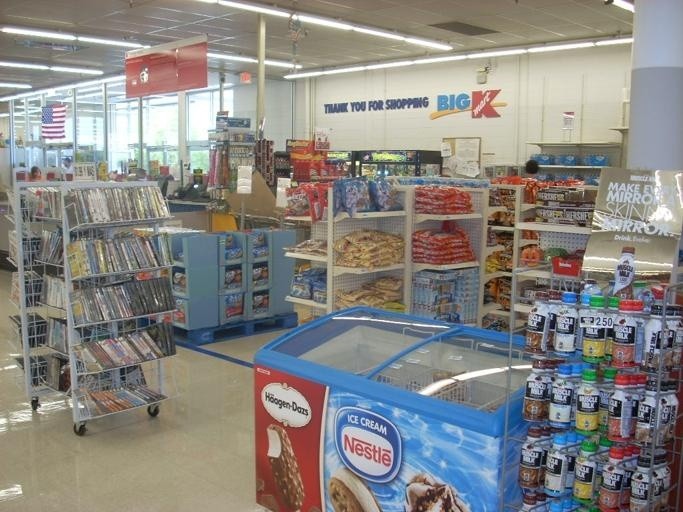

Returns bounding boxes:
[62,158,73,181]
[30,166,41,182]
[525,160,539,178]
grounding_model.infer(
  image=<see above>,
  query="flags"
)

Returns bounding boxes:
[41,105,66,139]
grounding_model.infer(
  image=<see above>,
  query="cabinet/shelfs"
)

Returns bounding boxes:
[510,186,598,332]
[500,263,682,512]
[5,182,179,437]
[526,141,624,187]
[284,185,490,328]
[483,179,516,333]
[208,128,274,192]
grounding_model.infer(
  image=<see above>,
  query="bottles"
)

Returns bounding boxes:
[517,247,683,511]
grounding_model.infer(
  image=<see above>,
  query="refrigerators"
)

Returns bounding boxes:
[252,305,550,512]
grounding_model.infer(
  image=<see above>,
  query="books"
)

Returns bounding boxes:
[5,185,176,417]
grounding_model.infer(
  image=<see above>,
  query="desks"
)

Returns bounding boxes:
[162,228,298,346]
